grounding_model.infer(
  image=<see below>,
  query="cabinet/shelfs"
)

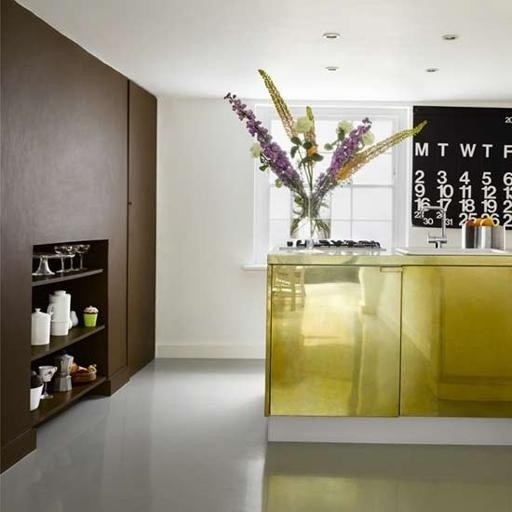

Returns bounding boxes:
[30,239,111,454]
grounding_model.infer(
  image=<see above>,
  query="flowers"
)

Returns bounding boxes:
[222,69,427,237]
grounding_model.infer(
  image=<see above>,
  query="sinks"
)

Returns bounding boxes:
[395,247,512,256]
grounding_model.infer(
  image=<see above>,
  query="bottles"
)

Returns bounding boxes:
[31,289,72,347]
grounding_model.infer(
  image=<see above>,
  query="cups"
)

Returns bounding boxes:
[83,311,98,327]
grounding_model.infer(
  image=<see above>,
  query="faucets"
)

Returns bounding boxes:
[420,206,446,237]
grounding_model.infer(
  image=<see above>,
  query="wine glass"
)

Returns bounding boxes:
[38,365,58,400]
[54,243,92,274]
